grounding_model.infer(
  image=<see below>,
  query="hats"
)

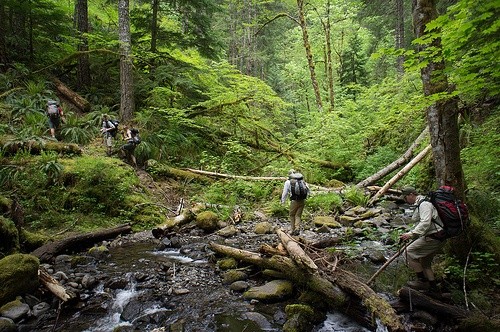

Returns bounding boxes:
[288,169,296,175]
[397,186,418,195]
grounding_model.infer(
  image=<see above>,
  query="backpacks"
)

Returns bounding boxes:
[416,186,470,240]
[105,120,119,137]
[48,101,59,117]
[128,129,141,144]
[288,173,308,201]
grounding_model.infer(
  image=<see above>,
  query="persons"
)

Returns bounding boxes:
[100,115,115,154]
[120,126,140,167]
[399,188,445,288]
[281,168,310,236]
[46,101,63,138]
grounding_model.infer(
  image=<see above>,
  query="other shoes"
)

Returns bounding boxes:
[105,153,112,157]
[407,276,430,290]
[294,230,299,236]
[417,277,442,293]
[289,231,295,235]
[122,159,128,162]
